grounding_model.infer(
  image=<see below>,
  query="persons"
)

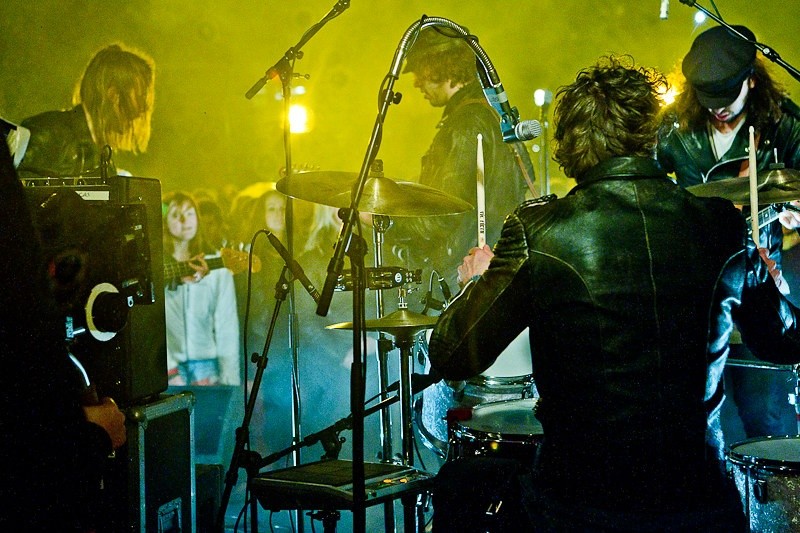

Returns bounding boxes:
[154,170,575,440]
[390,21,540,310]
[426,49,800,533]
[647,23,800,312]
[1,114,129,533]
[12,43,210,292]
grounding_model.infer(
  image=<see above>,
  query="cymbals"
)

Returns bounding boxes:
[682,163,800,204]
[324,308,442,343]
[275,171,475,217]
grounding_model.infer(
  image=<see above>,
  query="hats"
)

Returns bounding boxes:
[402,25,470,73]
[682,25,757,110]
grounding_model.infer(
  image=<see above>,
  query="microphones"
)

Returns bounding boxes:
[502,120,542,143]
[264,229,320,305]
[540,90,552,122]
[660,0,669,20]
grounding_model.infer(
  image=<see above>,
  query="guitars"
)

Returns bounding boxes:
[163,248,262,282]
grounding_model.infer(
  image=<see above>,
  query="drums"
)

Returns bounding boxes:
[444,397,548,472]
[413,320,545,450]
[725,434,800,532]
